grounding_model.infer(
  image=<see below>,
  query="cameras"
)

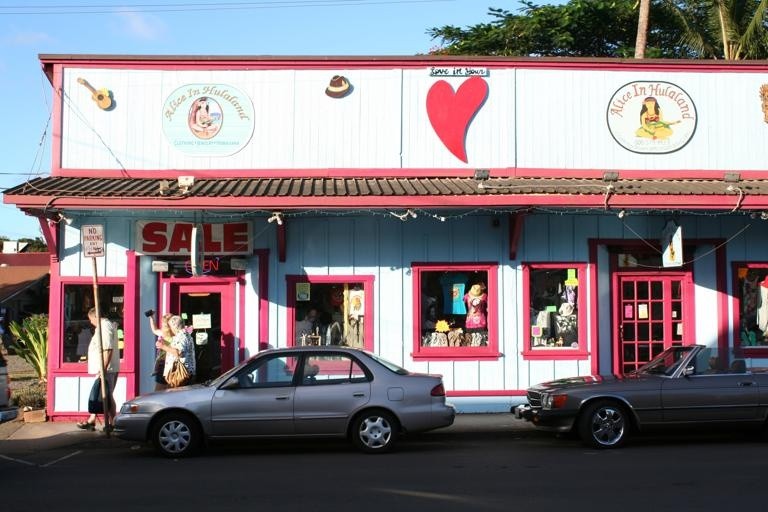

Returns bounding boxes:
[144,309,152,317]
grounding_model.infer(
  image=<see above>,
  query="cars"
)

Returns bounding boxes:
[111,345,455,459]
[514,345,768,450]
[0,350,17,421]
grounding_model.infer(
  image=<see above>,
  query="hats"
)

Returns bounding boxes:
[468,284,484,300]
[558,302,575,316]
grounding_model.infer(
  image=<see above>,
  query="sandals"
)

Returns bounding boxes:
[421,331,488,348]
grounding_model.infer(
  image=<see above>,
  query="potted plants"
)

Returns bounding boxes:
[14,390,47,424]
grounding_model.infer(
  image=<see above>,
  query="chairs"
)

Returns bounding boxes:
[726,361,743,376]
[302,364,319,385]
[707,357,720,374]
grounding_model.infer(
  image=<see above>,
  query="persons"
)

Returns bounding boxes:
[634,95,673,141]
[667,235,674,261]
[76,308,122,433]
[146,311,176,393]
[154,314,196,387]
[461,283,487,333]
[566,285,575,302]
[190,99,218,134]
[424,305,438,329]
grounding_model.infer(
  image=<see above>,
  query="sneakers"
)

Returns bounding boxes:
[77,420,96,432]
[98,423,114,433]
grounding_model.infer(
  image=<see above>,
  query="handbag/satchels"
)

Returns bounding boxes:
[165,349,193,388]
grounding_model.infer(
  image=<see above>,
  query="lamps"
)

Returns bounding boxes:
[725,173,740,182]
[604,173,618,181]
[177,176,195,194]
[475,170,488,179]
